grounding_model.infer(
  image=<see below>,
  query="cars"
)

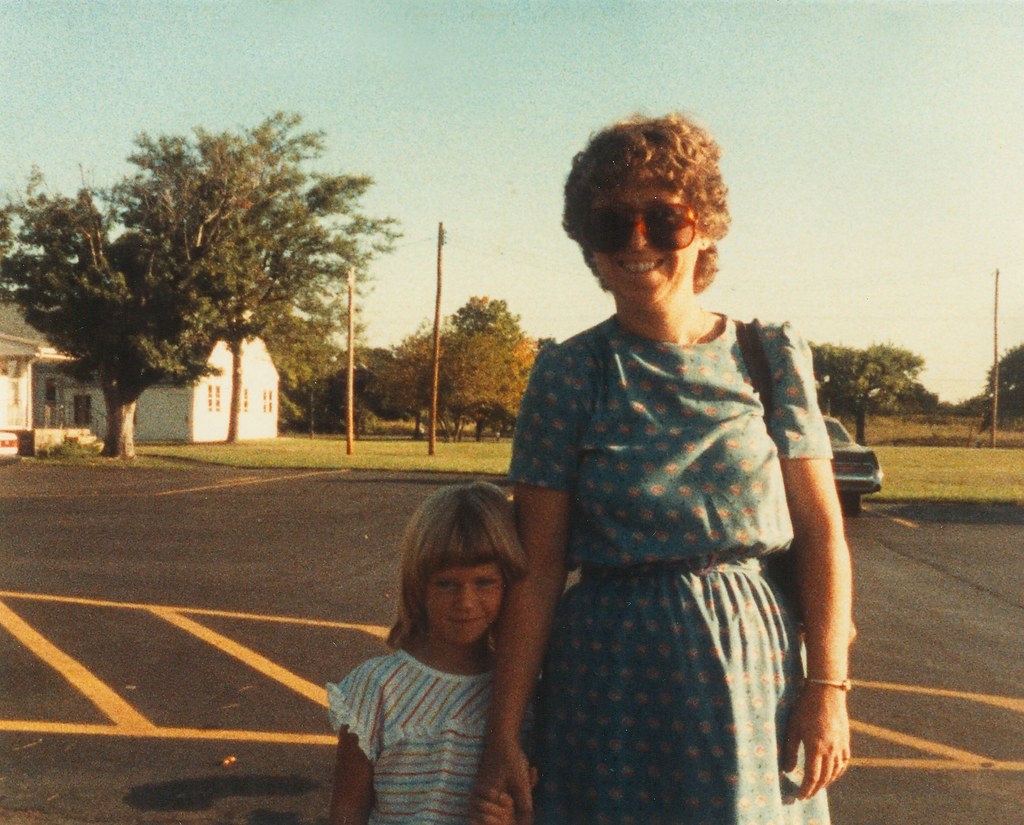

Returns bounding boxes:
[823,415,884,511]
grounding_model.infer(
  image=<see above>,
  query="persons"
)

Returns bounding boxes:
[327,481,538,825]
[479,112,852,825]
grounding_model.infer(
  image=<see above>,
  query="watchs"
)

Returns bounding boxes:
[806,675,855,693]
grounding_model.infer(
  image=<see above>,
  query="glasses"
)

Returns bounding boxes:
[579,202,700,252]
